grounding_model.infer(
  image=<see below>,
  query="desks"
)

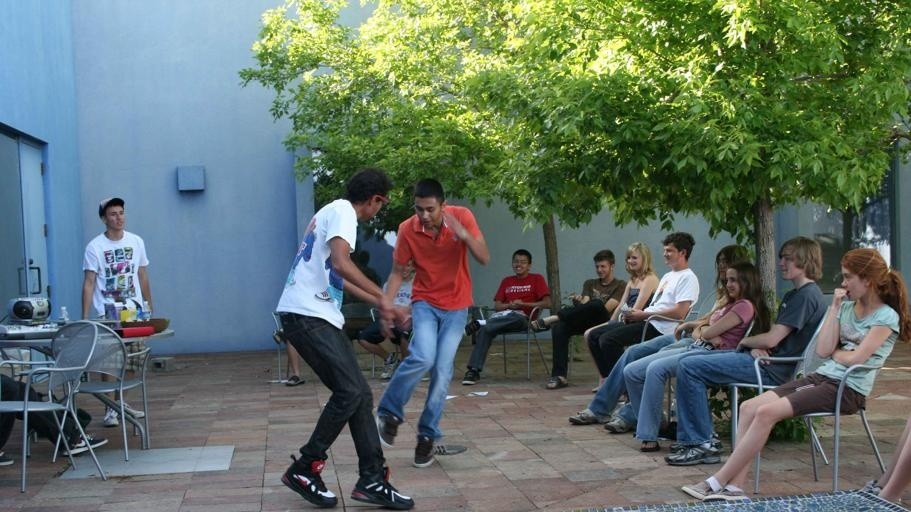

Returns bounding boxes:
[0,329,177,458]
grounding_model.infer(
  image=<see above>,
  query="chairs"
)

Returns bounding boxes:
[618,287,717,342]
[370,307,413,376]
[479,305,549,380]
[0,314,66,400]
[754,364,886,494]
[271,311,290,383]
[50,320,152,461]
[666,316,755,425]
[724,306,828,464]
[0,322,106,493]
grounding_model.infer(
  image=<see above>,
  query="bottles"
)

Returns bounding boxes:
[120,306,129,321]
[60,305,68,320]
[143,302,152,321]
[56,316,66,327]
[115,302,124,329]
[104,302,115,327]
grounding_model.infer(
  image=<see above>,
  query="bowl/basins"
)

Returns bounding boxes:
[121,317,170,333]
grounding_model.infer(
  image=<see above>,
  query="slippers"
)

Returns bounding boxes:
[531,319,550,332]
[547,377,568,389]
[640,441,660,452]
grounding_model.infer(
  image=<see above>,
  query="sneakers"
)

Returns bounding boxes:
[664,442,720,465]
[104,405,119,426]
[862,480,882,496]
[62,434,108,456]
[287,376,305,385]
[568,411,608,424]
[281,454,337,507]
[605,415,632,433]
[351,467,414,509]
[121,403,145,418]
[273,330,282,344]
[462,370,480,384]
[377,410,401,448]
[0,452,15,466]
[413,436,435,467]
[702,489,746,500]
[380,359,400,379]
[681,481,715,500]
[464,318,480,336]
[670,434,723,453]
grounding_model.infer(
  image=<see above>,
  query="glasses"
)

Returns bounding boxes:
[513,259,528,263]
[380,196,390,204]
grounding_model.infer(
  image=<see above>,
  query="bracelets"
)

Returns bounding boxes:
[700,323,709,330]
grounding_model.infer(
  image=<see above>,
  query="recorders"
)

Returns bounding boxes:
[6,278,51,319]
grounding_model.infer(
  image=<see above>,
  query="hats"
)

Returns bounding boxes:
[99,197,124,214]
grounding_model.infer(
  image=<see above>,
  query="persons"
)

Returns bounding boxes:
[461,249,553,385]
[623,261,771,452]
[860,414,911,505]
[82,197,153,428]
[357,256,413,380]
[347,250,381,302]
[584,241,659,402]
[275,166,415,510]
[529,250,626,390]
[682,249,911,501]
[568,244,750,434]
[376,178,490,468]
[587,231,700,404]
[272,328,305,386]
[664,236,829,466]
[0,373,109,466]
